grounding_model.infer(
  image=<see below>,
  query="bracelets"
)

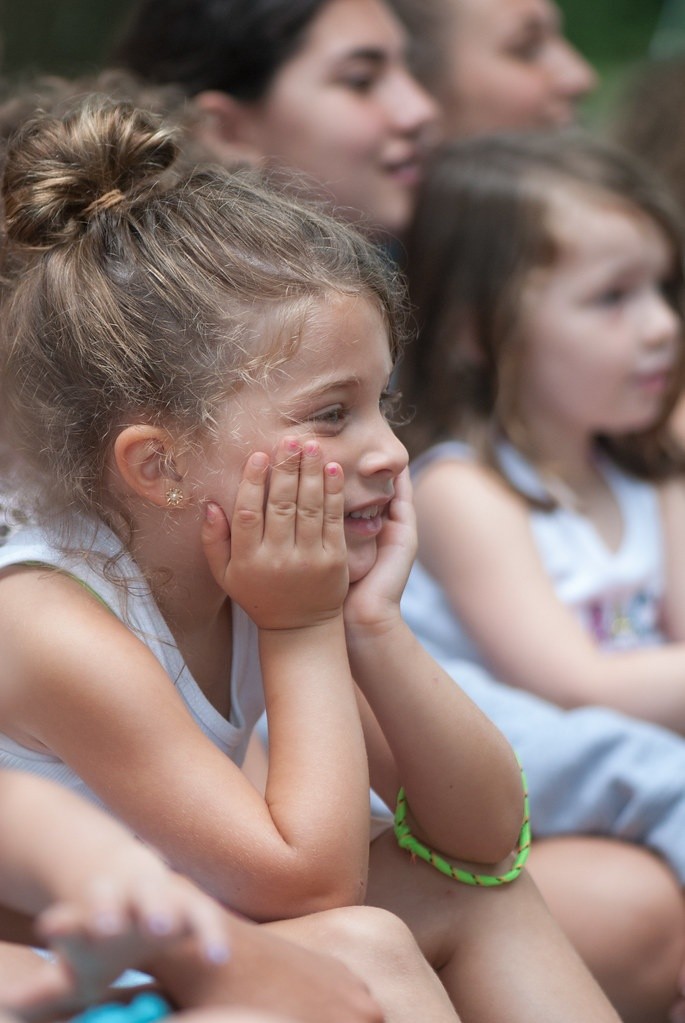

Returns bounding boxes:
[393,748,531,888]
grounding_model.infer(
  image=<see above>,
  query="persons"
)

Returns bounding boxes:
[0,0,685,1023]
